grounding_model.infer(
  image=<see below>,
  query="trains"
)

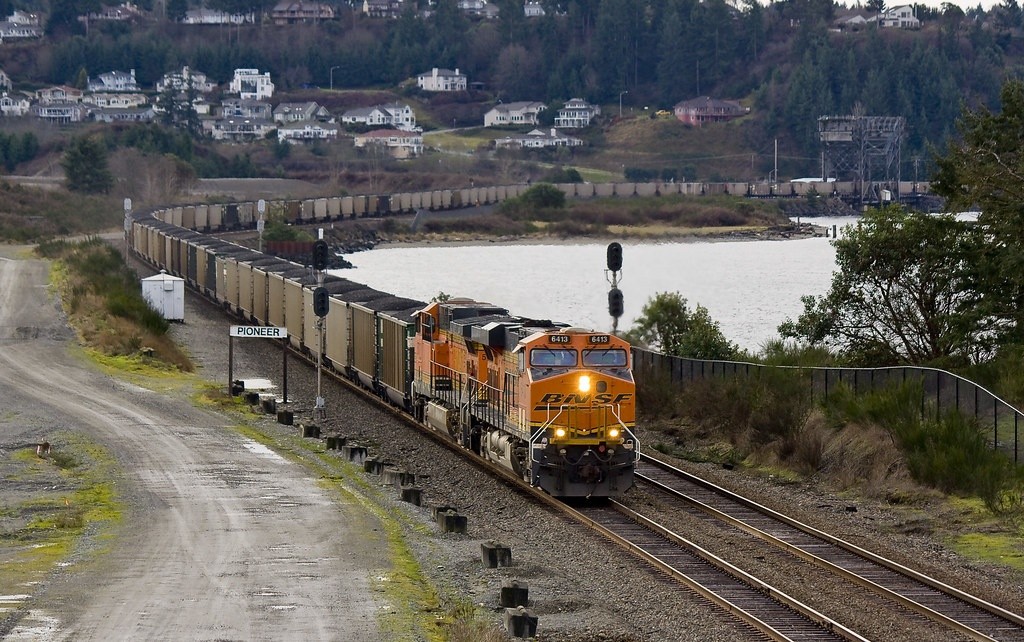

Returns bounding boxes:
[123,177,930,496]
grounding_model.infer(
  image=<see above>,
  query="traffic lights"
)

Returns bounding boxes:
[608,288,624,317]
[606,242,623,273]
[314,238,329,271]
[313,287,331,317]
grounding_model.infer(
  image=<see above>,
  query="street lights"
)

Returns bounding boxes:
[330,66,340,90]
[619,90,629,119]
